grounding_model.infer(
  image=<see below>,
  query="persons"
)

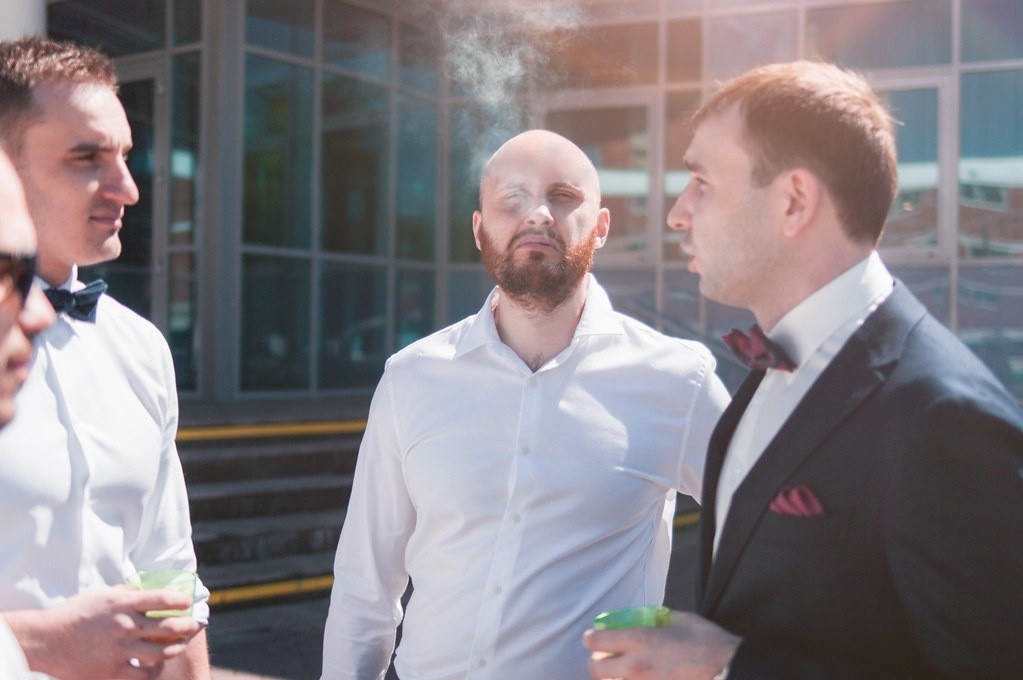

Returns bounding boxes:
[581,59,1023,680]
[0,34,210,680]
[319,128,732,680]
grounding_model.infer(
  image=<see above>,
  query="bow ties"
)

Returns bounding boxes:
[44,279,107,324]
[723,323,797,372]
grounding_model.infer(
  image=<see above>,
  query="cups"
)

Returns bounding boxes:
[138,568,197,645]
[592,605,671,664]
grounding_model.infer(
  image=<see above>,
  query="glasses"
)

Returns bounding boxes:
[0,254,36,306]
